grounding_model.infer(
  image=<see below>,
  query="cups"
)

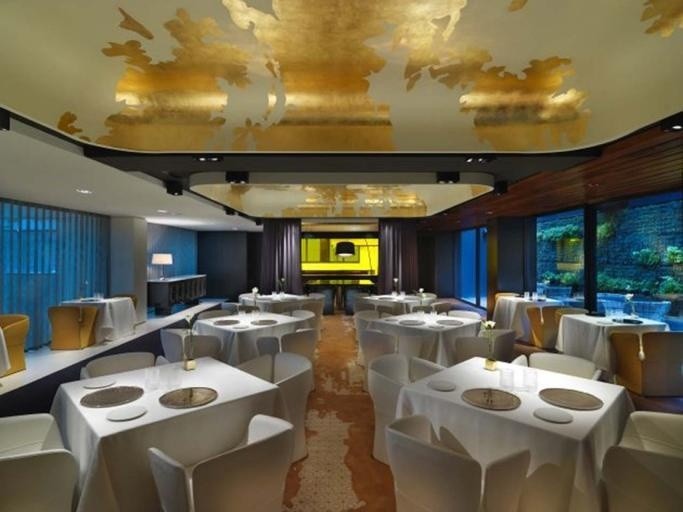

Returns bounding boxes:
[431,311,437,317]
[93,292,104,300]
[251,310,258,318]
[524,291,538,299]
[416,310,424,317]
[605,309,624,324]
[500,369,514,388]
[145,367,161,390]
[523,368,537,390]
[239,312,245,317]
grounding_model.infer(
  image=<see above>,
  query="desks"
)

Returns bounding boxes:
[147,275,207,315]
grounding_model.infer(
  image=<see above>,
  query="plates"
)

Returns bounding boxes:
[428,379,603,423]
[385,318,464,328]
[81,377,218,421]
[214,320,277,329]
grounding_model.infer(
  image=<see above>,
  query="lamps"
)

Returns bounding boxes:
[336,242,355,257]
[151,253,173,280]
